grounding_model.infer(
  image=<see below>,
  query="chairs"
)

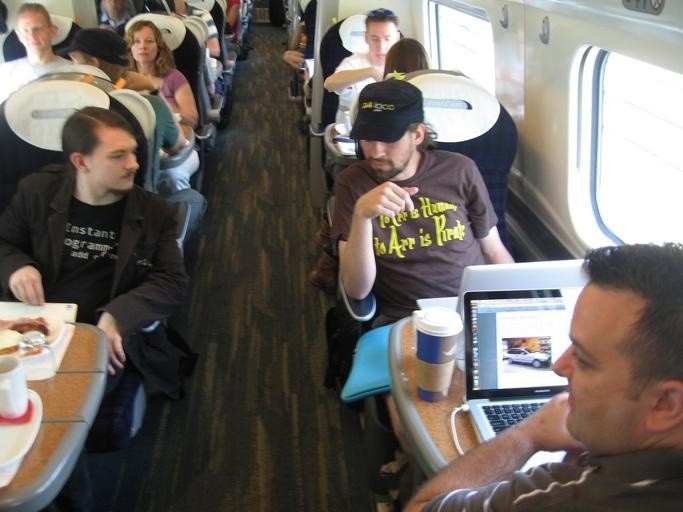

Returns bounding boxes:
[187,0,237,81]
[319,70,521,458]
[174,20,226,126]
[316,14,405,190]
[14,73,161,199]
[125,14,220,192]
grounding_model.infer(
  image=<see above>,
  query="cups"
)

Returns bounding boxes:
[1,356,26,420]
[414,308,463,403]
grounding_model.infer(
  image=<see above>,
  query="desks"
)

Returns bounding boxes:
[384,305,559,485]
[155,122,194,170]
[321,119,360,170]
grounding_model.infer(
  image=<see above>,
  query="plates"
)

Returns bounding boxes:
[0,389,43,489]
[0,311,66,361]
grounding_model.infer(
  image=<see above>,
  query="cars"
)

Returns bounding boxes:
[504,347,550,367]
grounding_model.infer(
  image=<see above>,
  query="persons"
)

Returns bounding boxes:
[173,0,221,59]
[332,79,516,324]
[323,8,404,95]
[0,4,73,108]
[403,241,683,512]
[209,1,253,128]
[124,22,199,131]
[383,38,432,82]
[65,28,208,260]
[100,0,136,39]
[282,1,317,104]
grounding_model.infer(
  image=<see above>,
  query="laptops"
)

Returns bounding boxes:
[459,286,587,472]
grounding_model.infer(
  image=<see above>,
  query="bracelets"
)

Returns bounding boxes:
[148,86,162,97]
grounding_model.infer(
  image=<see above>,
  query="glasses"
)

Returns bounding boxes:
[364,8,395,20]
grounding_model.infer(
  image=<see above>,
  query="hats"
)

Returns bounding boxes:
[351,78,425,144]
[52,29,133,68]
